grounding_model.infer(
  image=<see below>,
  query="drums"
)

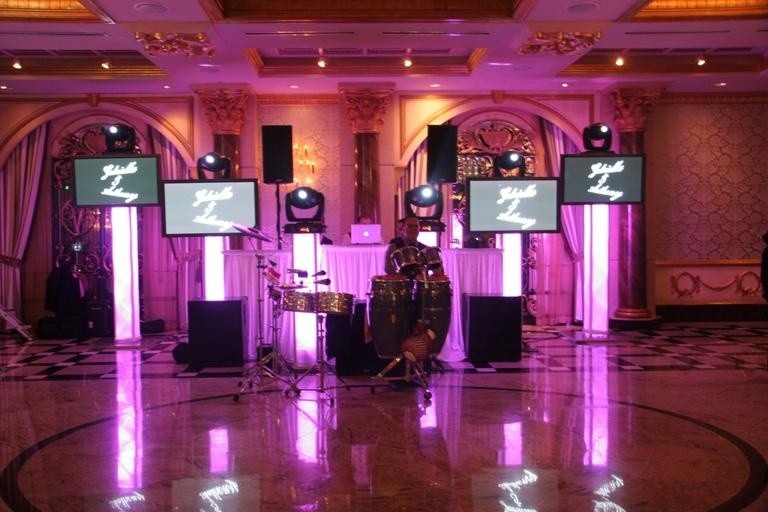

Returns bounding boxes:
[421,247,441,270]
[368,275,412,315]
[390,246,422,277]
[282,291,313,313]
[414,276,451,319]
[314,292,354,314]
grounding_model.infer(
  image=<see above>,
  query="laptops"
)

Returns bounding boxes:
[350,224,383,246]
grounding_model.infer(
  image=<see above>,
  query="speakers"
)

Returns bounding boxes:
[463,293,523,365]
[426,124,458,184]
[260,124,294,185]
[186,298,244,368]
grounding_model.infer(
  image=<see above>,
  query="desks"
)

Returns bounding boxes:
[223,246,505,371]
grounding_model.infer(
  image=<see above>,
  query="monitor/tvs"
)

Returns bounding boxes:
[464,176,566,232]
[72,154,161,208]
[159,178,258,237]
[559,153,646,206]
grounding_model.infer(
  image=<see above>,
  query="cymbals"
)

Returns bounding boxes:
[233,223,272,243]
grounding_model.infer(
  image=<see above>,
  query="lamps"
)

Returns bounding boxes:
[102,122,135,152]
[582,123,613,150]
[493,150,526,177]
[404,184,443,219]
[196,154,231,179]
[284,186,325,221]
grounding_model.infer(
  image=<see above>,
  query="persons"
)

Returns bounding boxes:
[343,215,374,245]
[314,219,333,244]
[384,217,445,274]
[396,218,405,238]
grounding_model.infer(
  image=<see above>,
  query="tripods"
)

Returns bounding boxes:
[236,291,298,387]
[232,257,301,402]
[370,353,432,400]
